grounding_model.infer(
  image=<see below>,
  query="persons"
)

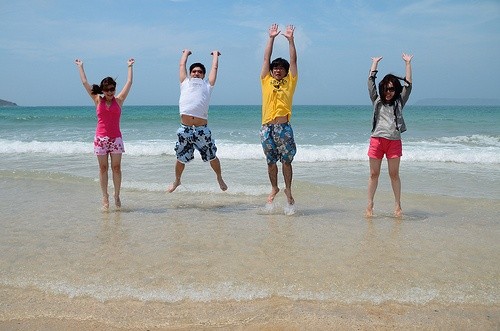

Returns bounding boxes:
[364,53,414,216]
[75,58,135,208]
[260,24,298,205]
[167,50,228,193]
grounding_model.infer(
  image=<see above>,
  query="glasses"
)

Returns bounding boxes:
[192,70,203,74]
[104,86,115,92]
[383,86,396,93]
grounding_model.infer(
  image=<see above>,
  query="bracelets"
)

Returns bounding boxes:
[128,65,133,67]
